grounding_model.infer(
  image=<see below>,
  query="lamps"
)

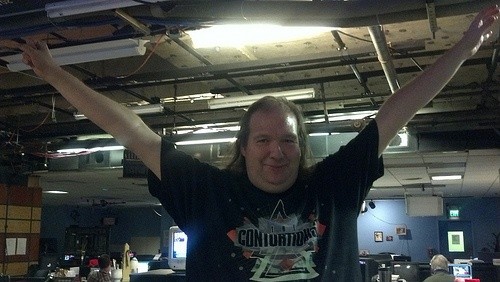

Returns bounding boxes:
[1,38,149,72]
[45,0,162,18]
[206,87,316,110]
[72,103,164,120]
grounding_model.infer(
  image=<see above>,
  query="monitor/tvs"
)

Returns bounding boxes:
[137,261,149,273]
[360,258,378,282]
[169,226,188,270]
[448,264,472,282]
[391,262,419,282]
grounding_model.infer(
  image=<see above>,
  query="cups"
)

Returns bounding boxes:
[378,268,391,282]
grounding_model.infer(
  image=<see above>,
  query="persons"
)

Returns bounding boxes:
[18,5,500,282]
[87,254,114,282]
[424,254,456,282]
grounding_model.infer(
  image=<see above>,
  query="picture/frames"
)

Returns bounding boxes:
[374,231,383,242]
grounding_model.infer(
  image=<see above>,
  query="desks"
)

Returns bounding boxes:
[129,269,186,282]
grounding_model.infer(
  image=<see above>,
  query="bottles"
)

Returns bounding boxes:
[130,252,138,274]
